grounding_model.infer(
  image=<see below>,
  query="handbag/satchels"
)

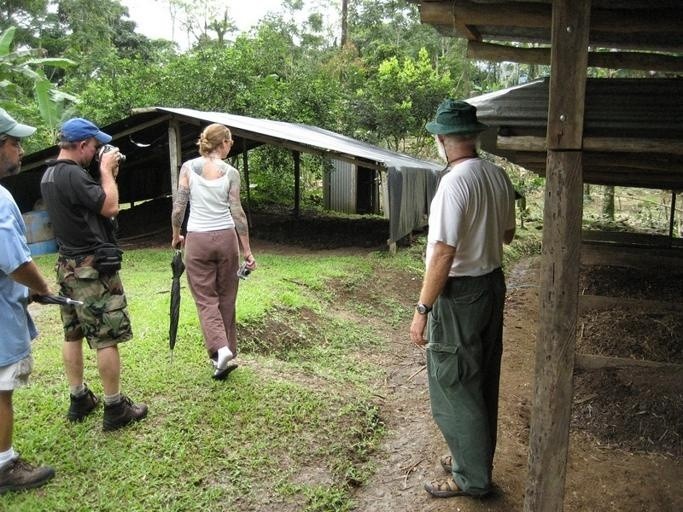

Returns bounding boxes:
[92,242,124,275]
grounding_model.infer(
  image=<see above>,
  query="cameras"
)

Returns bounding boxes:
[237,261,252,281]
[99,145,126,164]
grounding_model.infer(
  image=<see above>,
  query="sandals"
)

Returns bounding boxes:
[426,477,490,498]
[440,453,458,472]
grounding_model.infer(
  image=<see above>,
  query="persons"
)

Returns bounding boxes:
[0,104,55,493]
[409,99,516,500]
[168,123,258,379]
[38,117,147,433]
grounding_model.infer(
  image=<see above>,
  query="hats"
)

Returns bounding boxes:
[425,100,489,135]
[0,107,37,139]
[61,117,114,144]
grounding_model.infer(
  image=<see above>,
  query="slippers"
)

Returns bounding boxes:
[210,364,240,377]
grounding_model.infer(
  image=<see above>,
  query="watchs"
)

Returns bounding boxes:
[415,303,431,316]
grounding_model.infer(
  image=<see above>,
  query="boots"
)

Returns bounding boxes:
[67,389,102,421]
[0,457,55,492]
[102,396,148,431]
